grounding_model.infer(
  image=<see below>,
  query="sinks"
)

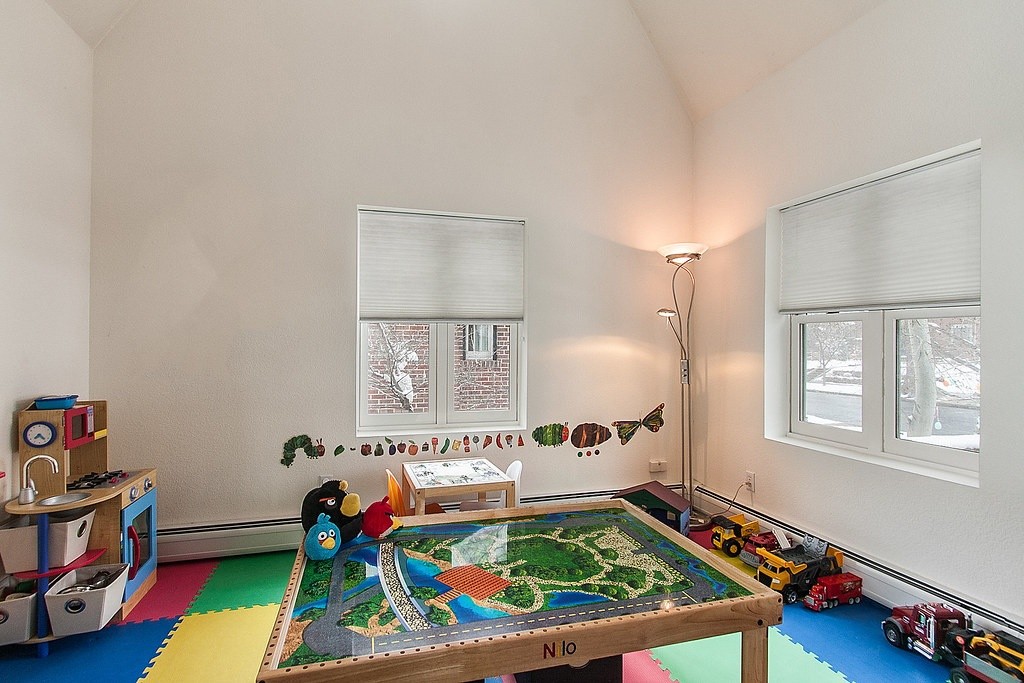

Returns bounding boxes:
[35,492,92,506]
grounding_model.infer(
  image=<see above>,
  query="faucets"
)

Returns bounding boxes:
[18,454,60,505]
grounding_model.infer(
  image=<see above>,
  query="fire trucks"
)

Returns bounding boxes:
[881,601,1024,683]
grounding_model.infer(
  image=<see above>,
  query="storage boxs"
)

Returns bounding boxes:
[0,504,97,574]
[43,563,130,638]
[0,575,38,645]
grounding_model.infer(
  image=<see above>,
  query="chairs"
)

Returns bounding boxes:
[460,459,522,512]
[386,469,445,517]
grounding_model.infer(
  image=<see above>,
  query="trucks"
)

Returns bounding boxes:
[802,572,863,612]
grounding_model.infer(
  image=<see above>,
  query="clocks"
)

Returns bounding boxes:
[23,421,56,448]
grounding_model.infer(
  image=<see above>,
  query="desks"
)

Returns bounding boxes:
[402,457,515,515]
[256,498,784,683]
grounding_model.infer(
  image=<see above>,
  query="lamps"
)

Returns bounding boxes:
[657,242,718,524]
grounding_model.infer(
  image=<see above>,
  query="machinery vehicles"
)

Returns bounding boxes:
[710,514,844,604]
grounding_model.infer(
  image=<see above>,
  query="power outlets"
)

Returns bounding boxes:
[745,470,755,493]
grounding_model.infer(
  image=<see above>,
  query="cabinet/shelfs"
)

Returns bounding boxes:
[4,401,157,657]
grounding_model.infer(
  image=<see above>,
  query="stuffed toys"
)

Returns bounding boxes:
[300,480,403,561]
